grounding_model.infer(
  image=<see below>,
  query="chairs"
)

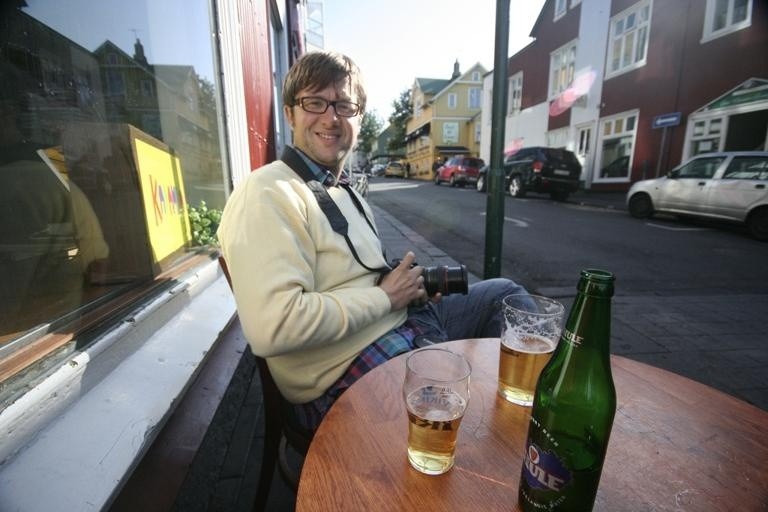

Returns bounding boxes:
[217,252,314,512]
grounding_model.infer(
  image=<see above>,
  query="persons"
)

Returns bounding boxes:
[215,52,552,437]
[0,88,87,339]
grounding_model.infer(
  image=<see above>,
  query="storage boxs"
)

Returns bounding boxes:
[63,120,194,285]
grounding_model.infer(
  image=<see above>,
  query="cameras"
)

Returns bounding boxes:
[387,258,470,298]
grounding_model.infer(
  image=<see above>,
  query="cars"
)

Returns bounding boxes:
[623,152,768,242]
[367,161,405,178]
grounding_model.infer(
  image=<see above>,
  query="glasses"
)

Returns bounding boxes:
[289,96,362,118]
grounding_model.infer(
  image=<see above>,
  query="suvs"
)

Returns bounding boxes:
[599,154,628,177]
[434,156,484,188]
[476,146,581,202]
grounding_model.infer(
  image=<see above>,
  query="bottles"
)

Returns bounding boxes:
[518,268,618,511]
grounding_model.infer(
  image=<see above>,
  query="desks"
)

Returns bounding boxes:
[296,336,766,512]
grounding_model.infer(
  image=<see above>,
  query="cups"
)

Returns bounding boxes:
[499,293,563,408]
[402,348,473,475]
